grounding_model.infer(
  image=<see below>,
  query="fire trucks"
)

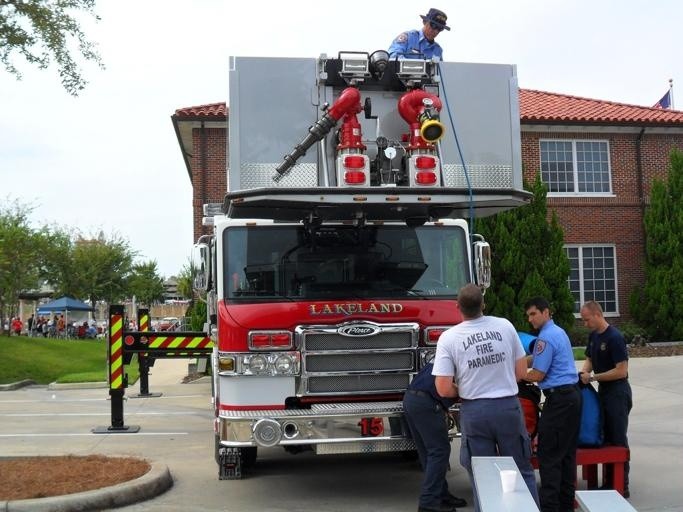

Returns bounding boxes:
[191,54,537,481]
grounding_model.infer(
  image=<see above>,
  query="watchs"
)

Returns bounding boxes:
[590,374,594,383]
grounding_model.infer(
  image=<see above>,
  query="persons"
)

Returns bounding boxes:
[0,311,162,341]
[404,359,469,512]
[577,301,634,499]
[384,7,451,60]
[521,296,584,512]
[431,283,541,512]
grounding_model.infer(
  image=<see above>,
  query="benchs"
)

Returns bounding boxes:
[531,442,630,508]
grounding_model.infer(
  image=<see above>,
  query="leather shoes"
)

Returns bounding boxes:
[417,505,456,512]
[444,492,468,509]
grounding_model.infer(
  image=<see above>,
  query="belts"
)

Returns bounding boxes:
[406,388,432,398]
[542,385,578,396]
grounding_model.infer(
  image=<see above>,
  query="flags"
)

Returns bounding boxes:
[653,91,670,109]
